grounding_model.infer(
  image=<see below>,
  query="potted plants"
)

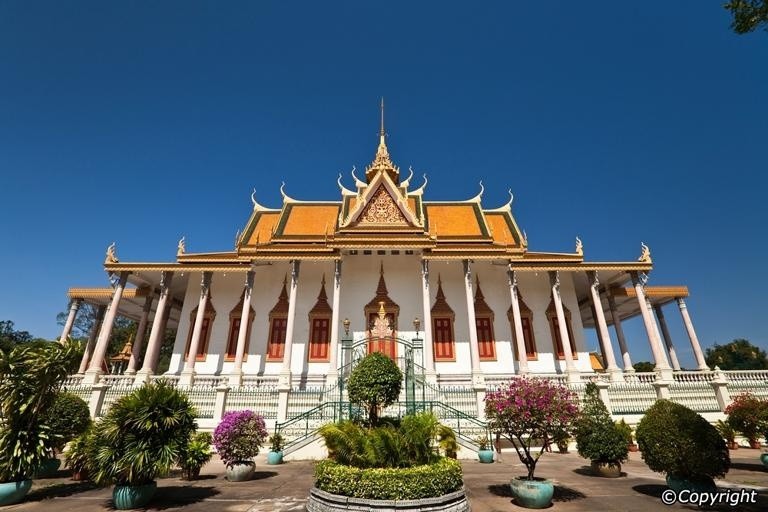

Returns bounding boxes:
[575,383,768,500]
[508,472,554,507]
[224,461,257,482]
[476,437,496,463]
[268,435,284,464]
[0,342,211,509]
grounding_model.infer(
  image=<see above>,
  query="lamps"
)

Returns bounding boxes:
[413,316,422,339]
[343,318,351,335]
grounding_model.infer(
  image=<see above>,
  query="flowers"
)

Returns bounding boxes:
[488,380,580,476]
[215,412,269,464]
